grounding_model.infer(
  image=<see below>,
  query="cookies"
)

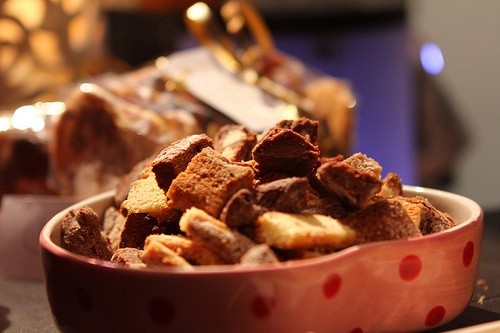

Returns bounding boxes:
[60,117,456,270]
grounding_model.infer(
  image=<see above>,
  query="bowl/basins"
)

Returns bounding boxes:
[38,180,485,333]
[0,194,96,282]
